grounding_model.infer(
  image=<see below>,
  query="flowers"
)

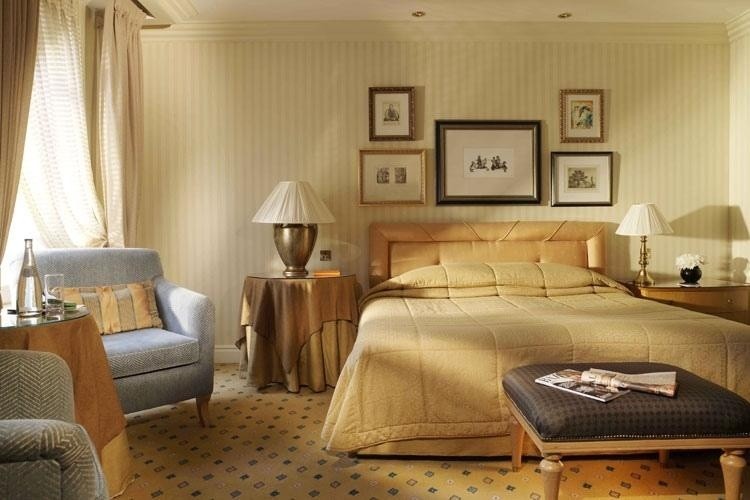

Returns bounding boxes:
[676,253,706,271]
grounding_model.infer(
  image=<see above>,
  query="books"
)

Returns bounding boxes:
[42,295,75,312]
[313,268,341,276]
[535,369,630,403]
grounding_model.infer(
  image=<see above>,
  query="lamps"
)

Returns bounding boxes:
[249,178,336,279]
[613,203,677,288]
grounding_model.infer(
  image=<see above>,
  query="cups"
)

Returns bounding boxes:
[42,274,65,322]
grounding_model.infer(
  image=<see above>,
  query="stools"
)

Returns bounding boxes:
[499,360,750,500]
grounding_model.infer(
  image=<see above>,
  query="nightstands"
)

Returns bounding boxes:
[616,276,749,326]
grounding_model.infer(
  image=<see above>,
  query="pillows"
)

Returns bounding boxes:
[48,280,166,337]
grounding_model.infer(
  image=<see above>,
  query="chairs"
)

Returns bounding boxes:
[0,346,109,500]
[29,244,218,430]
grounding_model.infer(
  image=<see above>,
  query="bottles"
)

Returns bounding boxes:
[16,239,43,319]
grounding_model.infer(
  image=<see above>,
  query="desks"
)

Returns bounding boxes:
[232,271,364,396]
[1,299,138,500]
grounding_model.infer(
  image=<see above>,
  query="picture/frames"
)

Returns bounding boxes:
[355,145,430,209]
[548,148,615,208]
[557,87,606,145]
[432,117,543,207]
[367,84,417,142]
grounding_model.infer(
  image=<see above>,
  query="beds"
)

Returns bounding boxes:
[320,216,749,500]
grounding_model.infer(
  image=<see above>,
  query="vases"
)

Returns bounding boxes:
[680,266,703,285]
